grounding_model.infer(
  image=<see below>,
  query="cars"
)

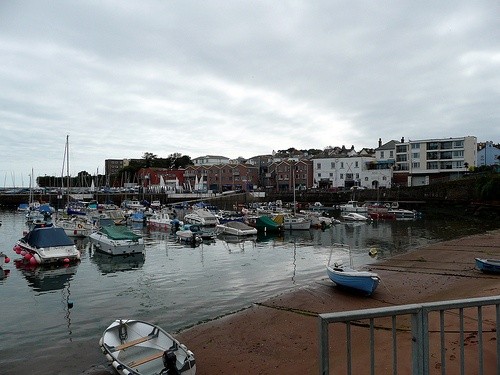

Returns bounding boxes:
[349,186,365,190]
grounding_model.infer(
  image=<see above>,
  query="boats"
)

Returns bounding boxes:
[178,222,216,240]
[325,243,382,297]
[271,214,311,230]
[216,221,258,236]
[0,252,10,267]
[12,226,80,266]
[473,257,500,274]
[176,229,203,245]
[99,319,197,375]
[14,133,424,234]
[243,215,282,233]
[88,224,145,256]
